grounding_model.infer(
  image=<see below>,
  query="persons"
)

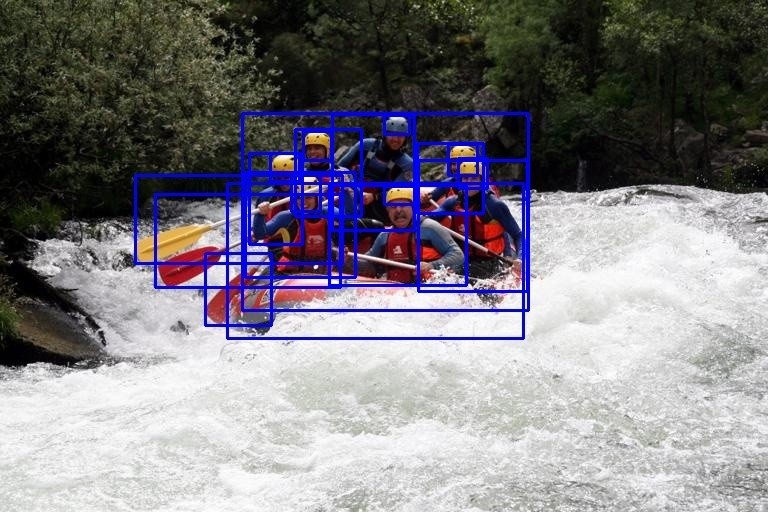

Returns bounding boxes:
[336,114,416,219]
[252,130,363,277]
[417,160,529,285]
[336,185,466,286]
[416,144,499,208]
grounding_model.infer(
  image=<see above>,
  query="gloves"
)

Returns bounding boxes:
[227,184,523,338]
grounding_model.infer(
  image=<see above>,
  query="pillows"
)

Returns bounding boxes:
[303,132,332,160]
[385,115,410,135]
[459,159,487,188]
[270,154,300,182]
[448,144,478,176]
[385,186,417,205]
[291,175,321,211]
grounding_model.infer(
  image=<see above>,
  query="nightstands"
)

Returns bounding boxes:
[205,253,272,325]
[136,176,342,264]
[156,195,340,288]
